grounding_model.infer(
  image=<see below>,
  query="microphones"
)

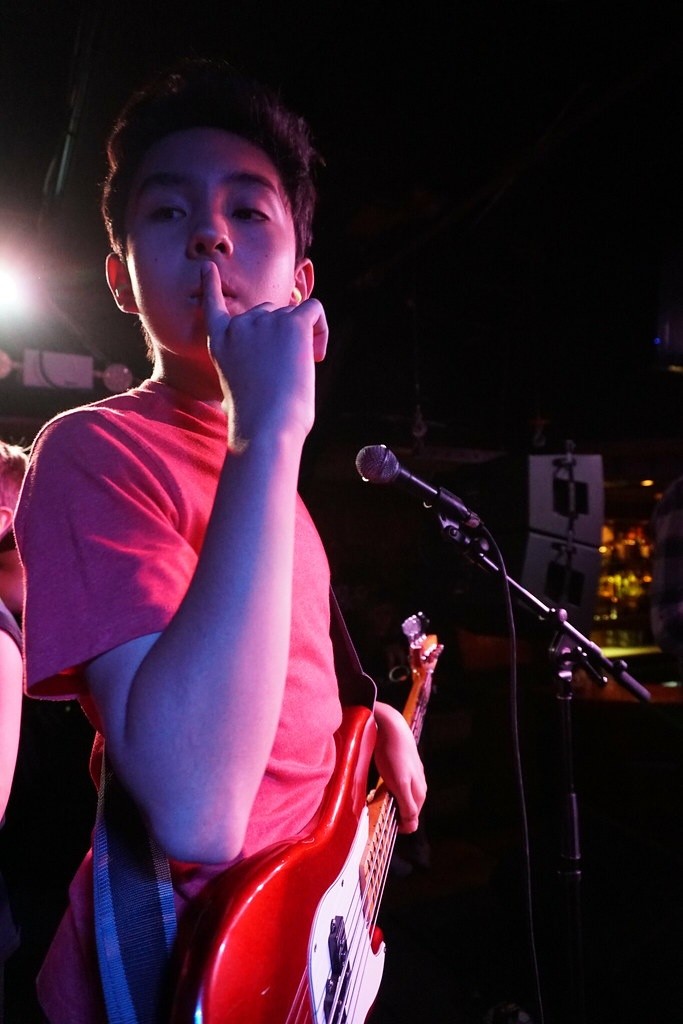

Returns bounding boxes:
[356,444,490,534]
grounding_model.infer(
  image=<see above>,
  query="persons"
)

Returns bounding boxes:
[652,476,683,681]
[0,443,28,819]
[13,69,428,1024]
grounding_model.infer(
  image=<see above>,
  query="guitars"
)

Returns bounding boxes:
[170,611,443,1024]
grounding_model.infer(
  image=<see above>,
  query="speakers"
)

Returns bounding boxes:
[512,454,604,641]
